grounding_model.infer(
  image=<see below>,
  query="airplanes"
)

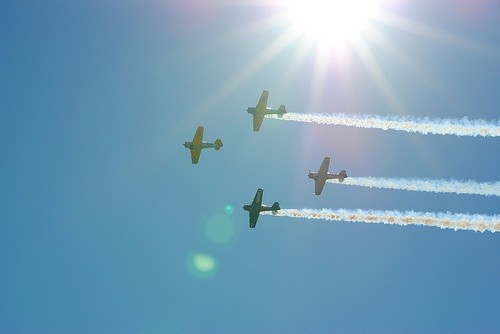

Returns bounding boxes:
[308,157,347,194]
[184,125,223,164]
[248,90,287,132]
[242,187,281,228]
